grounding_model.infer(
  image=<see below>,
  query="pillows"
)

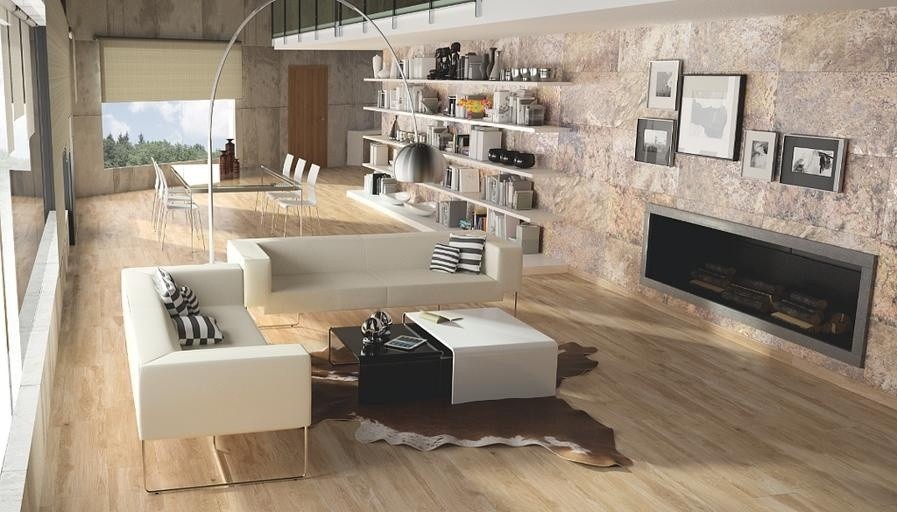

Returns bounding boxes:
[155,268,224,349]
[430,233,486,274]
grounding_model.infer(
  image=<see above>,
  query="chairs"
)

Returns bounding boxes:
[255,153,324,238]
[149,157,206,258]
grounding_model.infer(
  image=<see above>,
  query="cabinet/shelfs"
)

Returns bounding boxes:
[346,78,572,276]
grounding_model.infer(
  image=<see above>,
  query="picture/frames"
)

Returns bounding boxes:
[634,60,849,192]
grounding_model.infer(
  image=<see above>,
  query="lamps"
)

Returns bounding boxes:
[206,0,448,264]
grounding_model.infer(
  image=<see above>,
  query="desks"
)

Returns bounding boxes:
[171,164,302,259]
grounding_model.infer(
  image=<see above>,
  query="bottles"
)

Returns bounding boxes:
[499,69,510,81]
[224,139,234,169]
[426,125,433,146]
[219,151,226,169]
[232,159,239,173]
[372,54,381,79]
[479,47,501,81]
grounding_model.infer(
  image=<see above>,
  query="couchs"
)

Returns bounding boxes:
[228,230,523,316]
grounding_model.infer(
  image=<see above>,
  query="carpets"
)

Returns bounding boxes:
[310,342,634,468]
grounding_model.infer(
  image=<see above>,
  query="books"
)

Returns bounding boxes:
[418,310,463,324]
[360,55,545,254]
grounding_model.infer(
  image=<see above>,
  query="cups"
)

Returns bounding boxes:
[455,56,464,79]
[510,68,554,83]
[396,130,425,146]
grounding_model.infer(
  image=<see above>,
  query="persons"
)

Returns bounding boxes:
[748,138,768,172]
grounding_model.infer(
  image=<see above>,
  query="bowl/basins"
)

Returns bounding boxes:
[378,192,410,206]
[403,201,438,217]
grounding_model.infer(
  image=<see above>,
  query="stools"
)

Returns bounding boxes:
[121,264,312,492]
